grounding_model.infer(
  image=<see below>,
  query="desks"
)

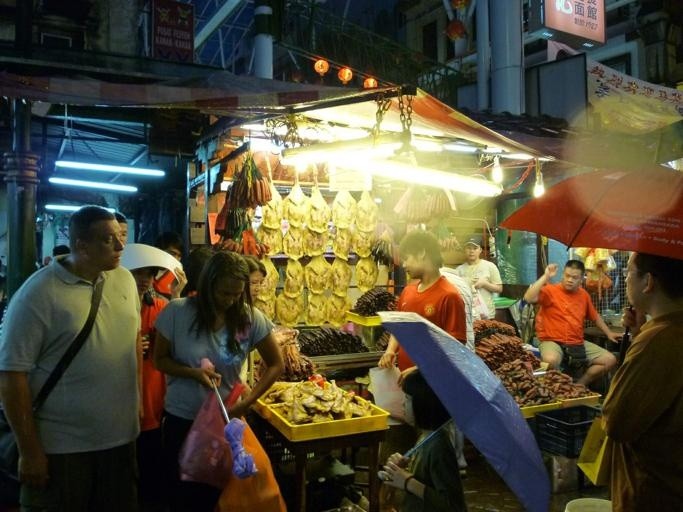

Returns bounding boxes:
[251,407,404,512]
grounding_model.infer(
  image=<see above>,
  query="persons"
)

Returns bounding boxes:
[438,256,477,479]
[600,249,682,511]
[377,362,468,511]
[378,230,467,511]
[455,237,505,320]
[521,260,628,392]
[0,204,284,512]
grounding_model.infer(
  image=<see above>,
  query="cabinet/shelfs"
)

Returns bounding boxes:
[186,140,394,351]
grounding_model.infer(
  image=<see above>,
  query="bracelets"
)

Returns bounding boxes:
[404,475,417,494]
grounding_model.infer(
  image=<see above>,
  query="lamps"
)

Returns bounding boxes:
[310,56,378,88]
[248,94,546,200]
[45,113,165,215]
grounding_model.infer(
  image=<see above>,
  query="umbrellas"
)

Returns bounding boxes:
[500,165,683,371]
[376,312,553,512]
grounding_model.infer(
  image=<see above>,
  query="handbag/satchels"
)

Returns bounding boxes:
[1,419,38,478]
[576,414,612,486]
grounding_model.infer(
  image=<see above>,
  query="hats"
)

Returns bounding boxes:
[463,236,482,247]
